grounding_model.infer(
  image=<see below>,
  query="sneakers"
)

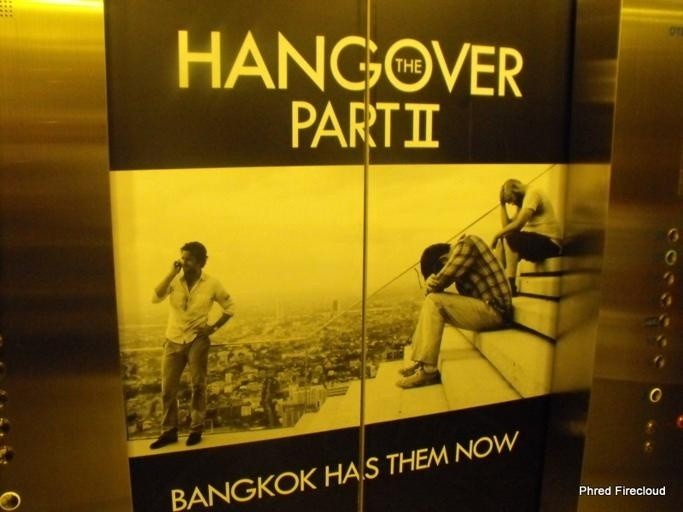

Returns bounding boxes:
[398,369,439,387]
[400,363,418,376]
[186,433,200,446]
[150,432,178,450]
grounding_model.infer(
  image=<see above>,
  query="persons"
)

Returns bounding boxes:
[149,240,236,448]
[492,176,560,295]
[396,234,513,388]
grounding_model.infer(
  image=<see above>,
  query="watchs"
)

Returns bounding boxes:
[213,324,218,332]
